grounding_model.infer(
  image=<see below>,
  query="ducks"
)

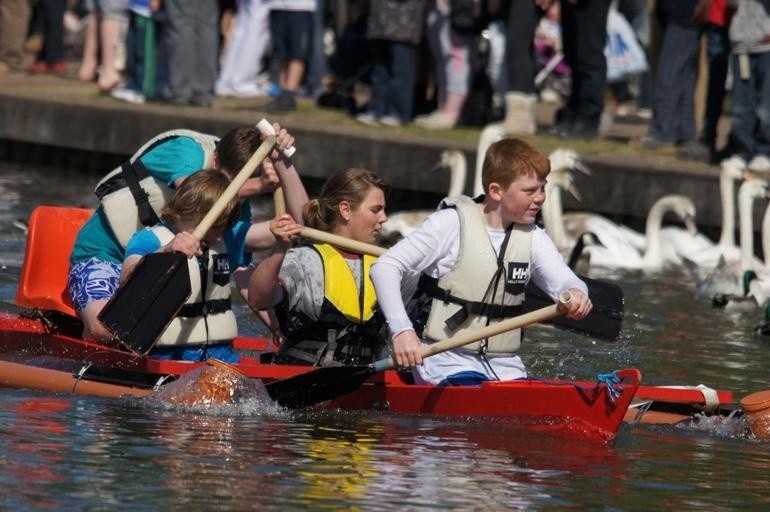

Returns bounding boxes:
[712,270,765,313]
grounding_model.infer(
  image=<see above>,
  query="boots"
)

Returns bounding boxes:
[492,95,536,135]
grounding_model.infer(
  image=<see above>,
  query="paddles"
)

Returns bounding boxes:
[282,213,624,342]
[97,118,297,360]
[264,291,574,410]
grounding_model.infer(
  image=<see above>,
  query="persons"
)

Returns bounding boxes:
[247,163,433,384]
[0,1,768,176]
[120,122,314,387]
[66,125,263,345]
[367,136,595,387]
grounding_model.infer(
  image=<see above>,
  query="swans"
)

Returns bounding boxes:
[376,123,770,305]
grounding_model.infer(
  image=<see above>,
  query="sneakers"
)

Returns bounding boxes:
[538,119,597,140]
[163,82,297,113]
[357,111,459,128]
[632,134,769,174]
[28,62,145,104]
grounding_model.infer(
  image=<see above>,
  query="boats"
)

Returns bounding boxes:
[0,203,643,444]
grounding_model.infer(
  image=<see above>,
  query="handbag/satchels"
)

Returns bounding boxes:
[367,0,426,46]
